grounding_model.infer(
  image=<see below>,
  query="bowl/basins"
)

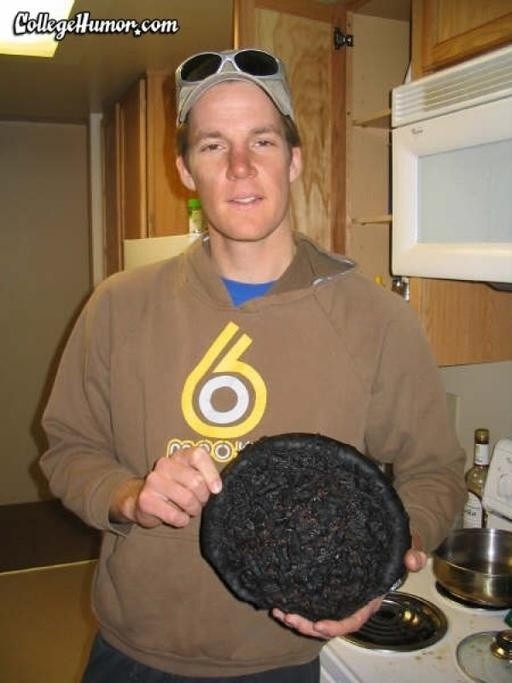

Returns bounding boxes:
[431,529,511,611]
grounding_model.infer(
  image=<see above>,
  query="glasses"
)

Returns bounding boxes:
[175,48,286,96]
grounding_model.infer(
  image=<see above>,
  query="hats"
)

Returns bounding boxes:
[176,50,301,144]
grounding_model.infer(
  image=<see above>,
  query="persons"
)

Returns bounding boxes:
[39,44,471,682]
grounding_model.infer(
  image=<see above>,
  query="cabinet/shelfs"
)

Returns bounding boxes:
[228,0,512,379]
[83,67,211,303]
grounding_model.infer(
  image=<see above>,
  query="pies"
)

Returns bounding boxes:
[200,433,411,622]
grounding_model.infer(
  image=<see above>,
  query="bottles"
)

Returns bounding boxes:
[463,428,495,528]
[186,196,205,244]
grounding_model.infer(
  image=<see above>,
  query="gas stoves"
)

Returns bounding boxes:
[323,556,511,683]
